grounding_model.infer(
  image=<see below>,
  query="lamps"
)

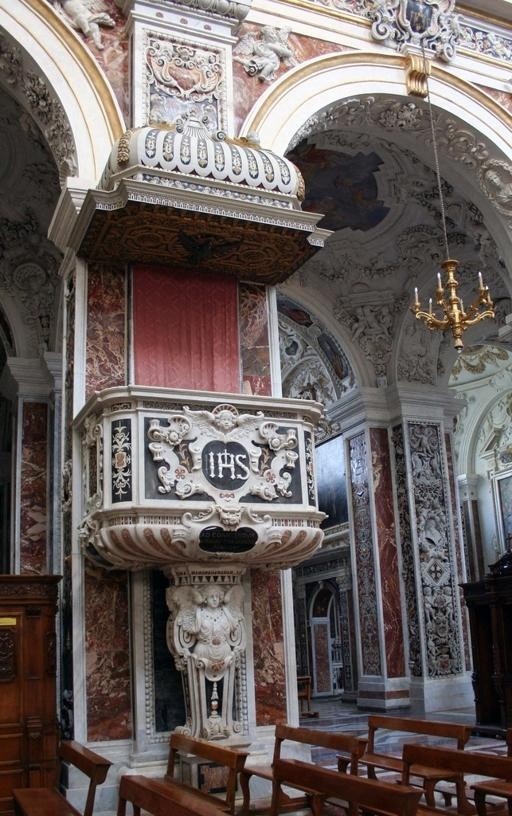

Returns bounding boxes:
[406,40,499,357]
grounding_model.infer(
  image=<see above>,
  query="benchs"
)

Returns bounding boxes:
[1,708,512,816]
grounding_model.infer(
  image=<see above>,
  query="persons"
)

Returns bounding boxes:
[422,469,434,486]
[417,501,432,530]
[193,584,239,663]
[415,428,435,455]
[351,304,394,345]
[422,587,436,624]
[59,0,117,50]
[215,409,238,434]
[440,587,453,626]
[247,22,291,81]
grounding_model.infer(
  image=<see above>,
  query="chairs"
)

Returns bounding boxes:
[291,675,312,712]
